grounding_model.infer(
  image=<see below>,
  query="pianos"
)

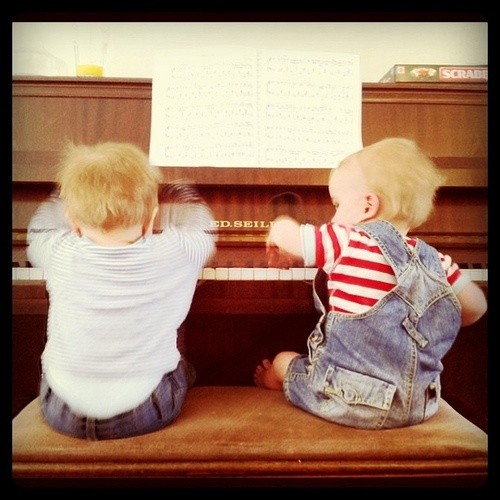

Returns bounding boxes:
[8,77,491,500]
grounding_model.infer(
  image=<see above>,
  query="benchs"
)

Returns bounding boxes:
[12,385,488,479]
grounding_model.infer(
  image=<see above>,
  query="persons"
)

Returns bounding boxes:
[252,137,487,431]
[25,133,218,441]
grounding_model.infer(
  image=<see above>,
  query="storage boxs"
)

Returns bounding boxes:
[379,64,488,80]
